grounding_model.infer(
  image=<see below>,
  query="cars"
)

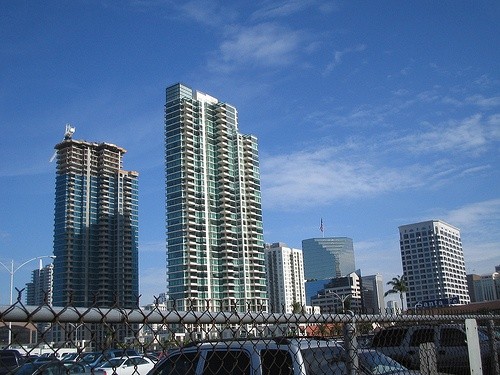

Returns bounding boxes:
[350,347,448,375]
[145,336,364,375]
[0,348,158,375]
[368,324,499,374]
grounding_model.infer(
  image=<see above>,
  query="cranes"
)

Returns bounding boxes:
[50,123,76,164]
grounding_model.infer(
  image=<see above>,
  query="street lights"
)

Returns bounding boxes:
[1,253,56,348]
[414,303,422,323]
[327,288,369,315]
[68,323,84,346]
